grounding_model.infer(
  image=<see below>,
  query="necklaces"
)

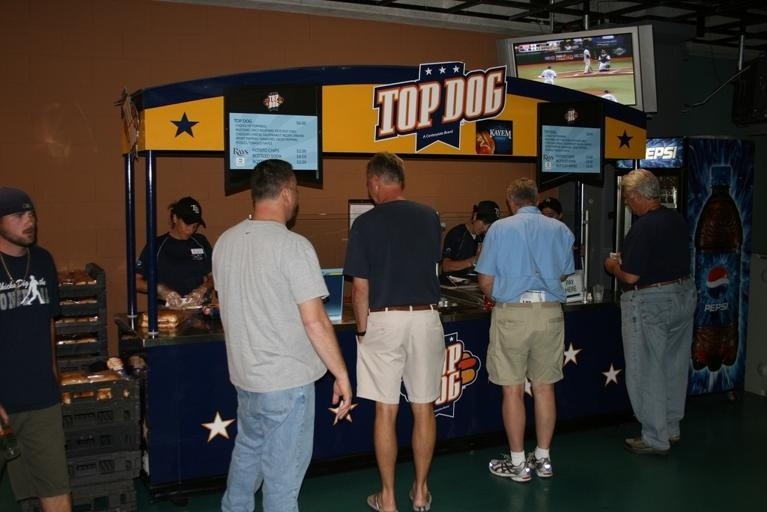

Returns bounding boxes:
[0,247,31,286]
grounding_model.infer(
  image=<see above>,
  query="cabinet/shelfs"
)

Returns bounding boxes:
[114,289,635,507]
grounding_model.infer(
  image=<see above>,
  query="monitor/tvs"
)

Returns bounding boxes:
[495,24,657,113]
[318,269,344,321]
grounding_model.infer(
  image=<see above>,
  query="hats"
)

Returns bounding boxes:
[0,187,34,216]
[171,197,206,228]
[473,200,500,224]
[538,197,562,215]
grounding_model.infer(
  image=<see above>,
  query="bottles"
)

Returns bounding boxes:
[691,166,742,371]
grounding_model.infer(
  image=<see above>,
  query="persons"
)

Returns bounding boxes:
[136,197,214,307]
[0,189,72,512]
[605,170,698,453]
[343,151,445,512]
[474,177,576,482]
[540,66,557,85]
[598,49,611,72]
[212,160,353,512]
[583,46,593,74]
[538,198,564,223]
[440,200,500,286]
[564,43,572,51]
[601,90,618,102]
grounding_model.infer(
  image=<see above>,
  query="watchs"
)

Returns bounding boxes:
[354,331,366,336]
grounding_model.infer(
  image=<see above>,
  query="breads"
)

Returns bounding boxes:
[58,271,96,285]
[58,367,129,401]
[56,334,98,344]
[56,314,100,324]
[59,296,98,306]
[141,310,185,329]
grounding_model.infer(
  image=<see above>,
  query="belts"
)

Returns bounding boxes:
[369,304,438,313]
[621,275,691,296]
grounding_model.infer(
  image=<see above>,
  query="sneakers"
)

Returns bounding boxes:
[489,453,532,482]
[623,435,669,453]
[527,450,553,478]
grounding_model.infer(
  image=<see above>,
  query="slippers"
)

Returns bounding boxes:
[367,491,399,512]
[408,487,432,512]
